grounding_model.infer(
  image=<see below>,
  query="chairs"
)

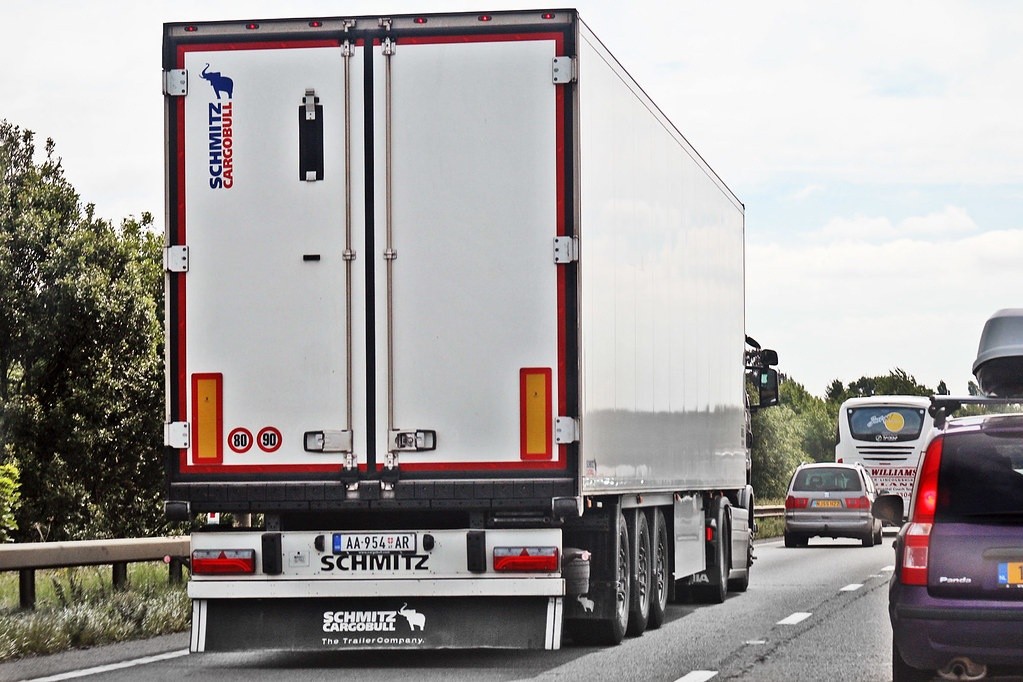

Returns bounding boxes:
[801,474,859,491]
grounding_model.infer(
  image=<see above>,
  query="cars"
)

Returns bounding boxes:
[889,395,1023,682]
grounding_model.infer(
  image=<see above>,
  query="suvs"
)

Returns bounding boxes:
[783,461,890,548]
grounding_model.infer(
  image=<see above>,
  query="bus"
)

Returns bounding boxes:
[834,395,953,527]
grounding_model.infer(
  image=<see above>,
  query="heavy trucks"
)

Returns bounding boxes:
[160,9,780,651]
